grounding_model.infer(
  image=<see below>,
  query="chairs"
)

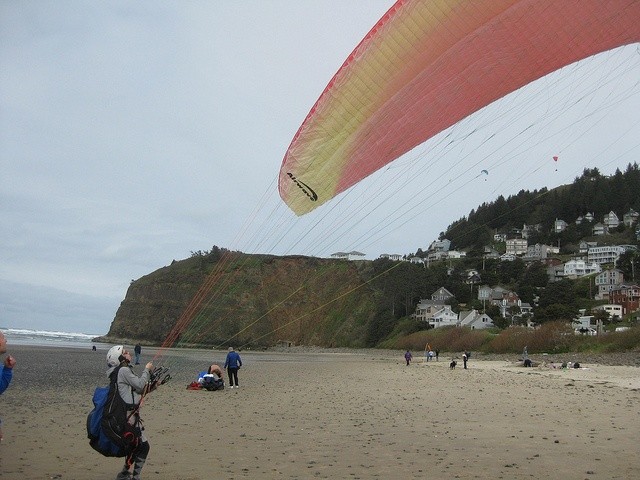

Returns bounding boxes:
[223,382,225,387]
[228,386,233,388]
[117,473,132,480]
[235,386,239,388]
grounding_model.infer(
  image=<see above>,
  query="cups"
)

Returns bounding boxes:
[123,352,128,355]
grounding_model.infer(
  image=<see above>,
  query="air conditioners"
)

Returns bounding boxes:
[450,360,457,370]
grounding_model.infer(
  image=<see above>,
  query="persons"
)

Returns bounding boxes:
[405,349,412,366]
[462,352,468,369]
[208,365,225,386]
[450,361,457,370]
[428,350,434,361]
[106,345,154,480]
[425,350,430,362]
[223,347,242,389]
[0,330,17,441]
[134,343,141,365]
[435,349,440,361]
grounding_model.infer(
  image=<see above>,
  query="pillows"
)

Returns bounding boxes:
[106,345,123,367]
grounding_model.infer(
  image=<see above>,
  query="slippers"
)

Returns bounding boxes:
[87,365,135,457]
